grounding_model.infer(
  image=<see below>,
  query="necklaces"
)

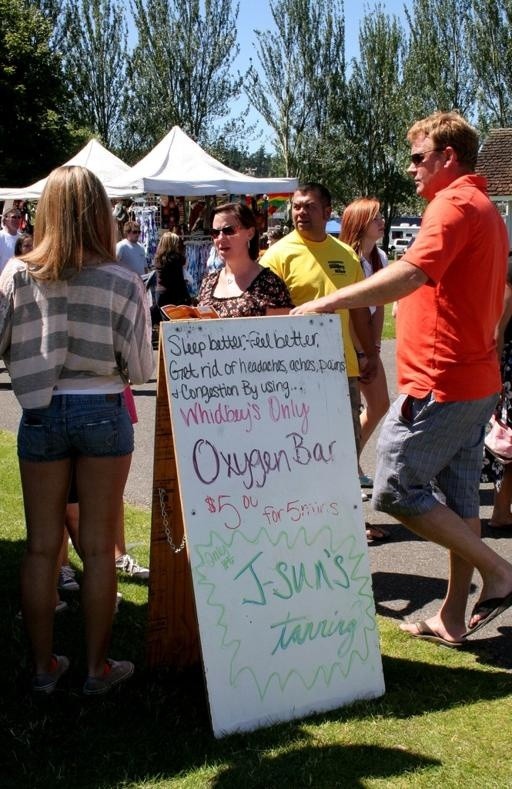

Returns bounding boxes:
[224,267,253,284]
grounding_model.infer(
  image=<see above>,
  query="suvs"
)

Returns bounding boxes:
[385,238,412,258]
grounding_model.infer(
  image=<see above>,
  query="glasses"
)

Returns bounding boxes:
[408,147,448,164]
[208,224,249,239]
[128,229,141,235]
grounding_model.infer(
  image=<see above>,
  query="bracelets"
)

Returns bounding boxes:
[357,351,365,360]
[375,343,381,352]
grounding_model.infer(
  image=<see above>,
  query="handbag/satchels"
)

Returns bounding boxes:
[120,378,140,426]
[485,415,511,464]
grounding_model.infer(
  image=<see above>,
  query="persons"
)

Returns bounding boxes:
[56,384,151,590]
[286,112,512,647]
[256,182,369,505]
[0,200,193,320]
[195,203,295,324]
[257,228,284,255]
[1,164,150,698]
[480,254,512,539]
[338,196,390,486]
[14,459,123,623]
[206,242,225,274]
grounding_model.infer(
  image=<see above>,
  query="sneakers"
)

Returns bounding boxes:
[32,652,70,691]
[116,554,151,579]
[54,567,79,591]
[359,469,374,502]
[114,590,125,617]
[82,655,136,693]
[15,598,67,619]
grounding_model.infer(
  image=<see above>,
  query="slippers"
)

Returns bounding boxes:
[400,615,467,650]
[486,518,511,530]
[367,519,392,549]
[460,583,512,639]
